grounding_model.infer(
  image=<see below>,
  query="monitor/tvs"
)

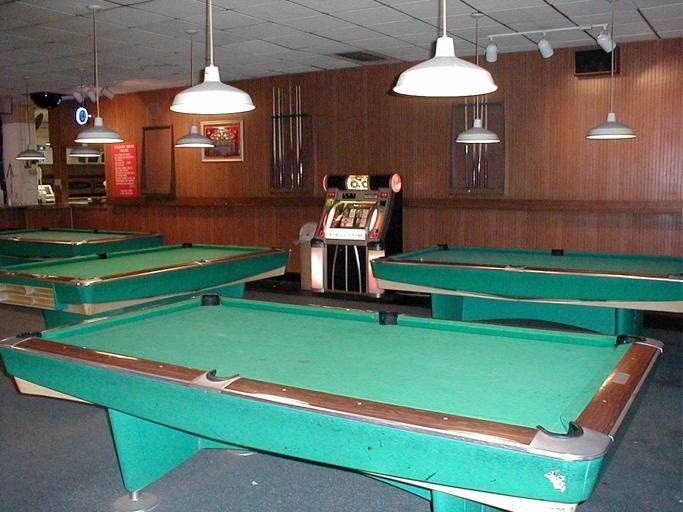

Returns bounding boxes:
[323,200,377,241]
[39,184,54,196]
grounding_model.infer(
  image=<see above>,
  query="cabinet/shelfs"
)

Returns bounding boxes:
[39,164,106,197]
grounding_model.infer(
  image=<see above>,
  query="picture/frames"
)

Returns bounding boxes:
[139,124,173,196]
[200,119,245,163]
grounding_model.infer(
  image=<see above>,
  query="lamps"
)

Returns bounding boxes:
[168,0,256,115]
[173,29,216,149]
[67,77,102,158]
[15,74,46,160]
[72,85,114,104]
[454,12,501,144]
[585,1,637,141]
[483,23,617,63]
[391,0,498,99]
[72,5,126,145]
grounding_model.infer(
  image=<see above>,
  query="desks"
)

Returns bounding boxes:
[370,244,683,336]
[1,290,669,512]
[2,227,164,256]
[3,243,292,328]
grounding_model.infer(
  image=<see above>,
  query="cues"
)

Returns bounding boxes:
[464,94,488,189]
[272,83,303,189]
